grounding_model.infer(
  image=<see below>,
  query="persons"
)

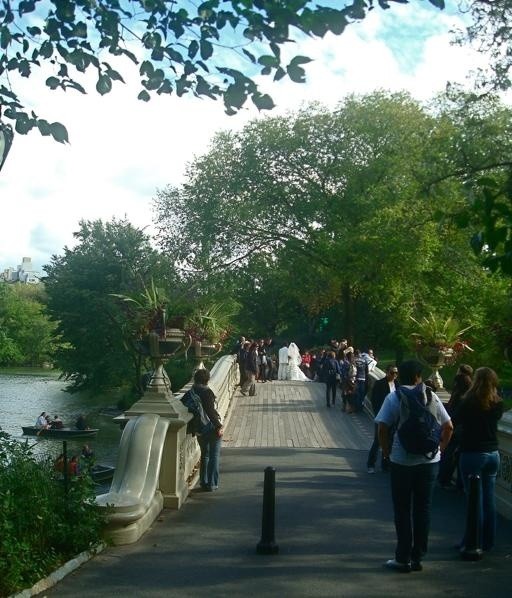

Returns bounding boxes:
[443,373,472,489]
[366,366,400,474]
[453,367,504,560]
[36,409,97,481]
[375,360,455,573]
[192,368,223,492]
[234,333,377,416]
[435,364,471,492]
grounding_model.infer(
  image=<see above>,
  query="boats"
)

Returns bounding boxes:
[21,423,101,439]
[78,460,116,483]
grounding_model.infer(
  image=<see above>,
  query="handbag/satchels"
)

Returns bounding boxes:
[328,368,339,379]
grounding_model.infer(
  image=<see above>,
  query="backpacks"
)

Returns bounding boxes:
[184,389,216,435]
[396,384,444,457]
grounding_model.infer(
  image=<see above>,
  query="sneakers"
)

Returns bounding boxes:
[385,556,412,573]
[367,467,376,475]
[241,390,258,397]
[458,541,495,554]
[409,560,424,572]
[201,482,219,493]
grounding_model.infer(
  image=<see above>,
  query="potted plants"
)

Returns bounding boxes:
[182,296,235,369]
[104,270,195,393]
[405,309,478,390]
